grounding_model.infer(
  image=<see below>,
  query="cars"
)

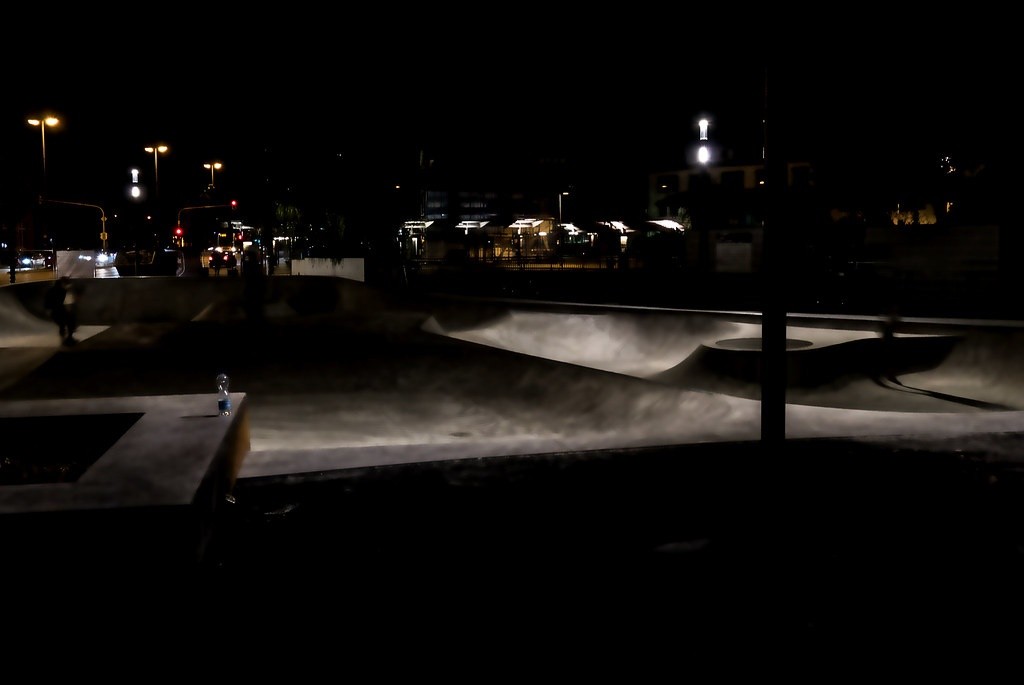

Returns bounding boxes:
[210,251,235,269]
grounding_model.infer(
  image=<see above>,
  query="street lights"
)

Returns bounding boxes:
[144,144,169,198]
[27,114,61,176]
[203,161,224,187]
[559,191,569,225]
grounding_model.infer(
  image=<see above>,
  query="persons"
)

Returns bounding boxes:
[47,276,81,344]
[213,255,222,276]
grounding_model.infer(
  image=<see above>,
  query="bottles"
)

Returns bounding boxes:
[218,382,232,416]
